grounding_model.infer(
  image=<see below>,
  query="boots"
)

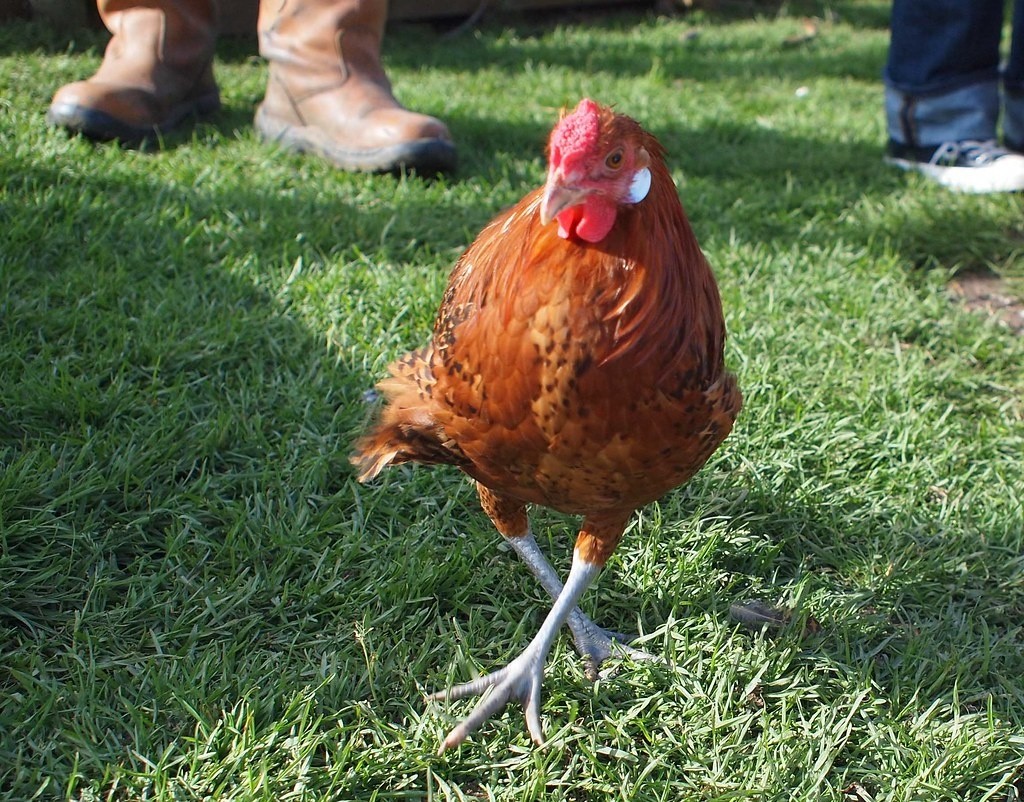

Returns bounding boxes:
[48,0,227,152]
[254,0,457,175]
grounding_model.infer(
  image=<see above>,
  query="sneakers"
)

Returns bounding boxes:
[882,135,1024,194]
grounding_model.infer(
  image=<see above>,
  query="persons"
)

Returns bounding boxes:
[48,0,458,178]
[880,0,1024,195]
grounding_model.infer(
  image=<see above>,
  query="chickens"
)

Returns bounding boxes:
[349,97,742,752]
